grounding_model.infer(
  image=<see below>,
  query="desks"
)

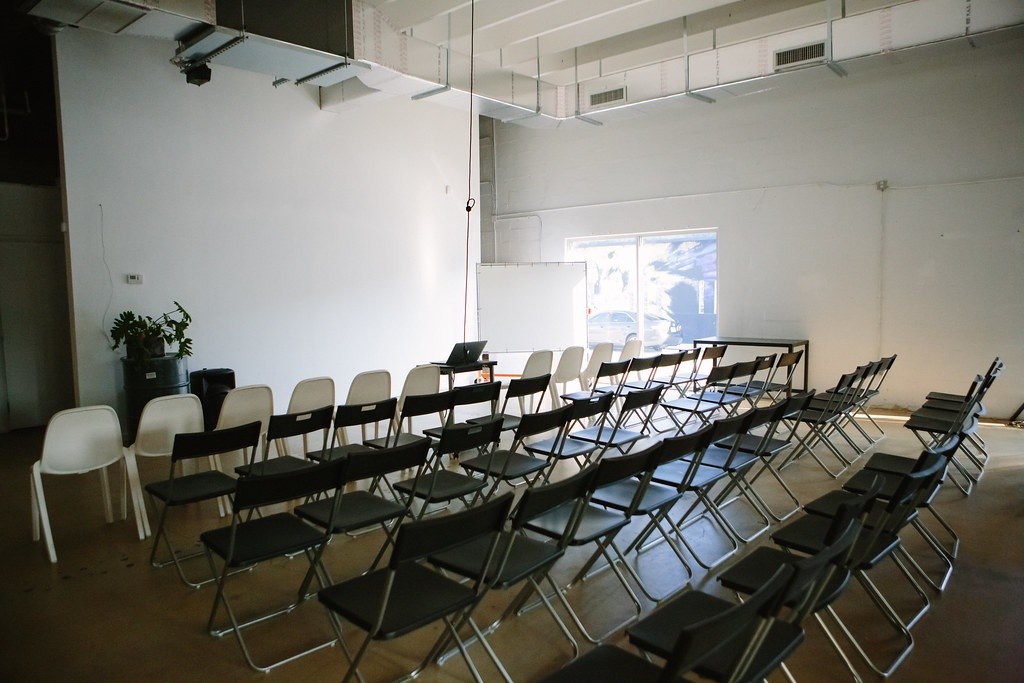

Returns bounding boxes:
[693,336,809,400]
[416,360,498,392]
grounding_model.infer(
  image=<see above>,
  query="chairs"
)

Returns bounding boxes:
[32,340,1004,683]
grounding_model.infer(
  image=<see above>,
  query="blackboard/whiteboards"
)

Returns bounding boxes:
[474,260,590,355]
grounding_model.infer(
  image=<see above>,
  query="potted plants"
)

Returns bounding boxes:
[110,300,193,360]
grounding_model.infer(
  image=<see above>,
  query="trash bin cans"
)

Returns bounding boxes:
[189,367,235,432]
